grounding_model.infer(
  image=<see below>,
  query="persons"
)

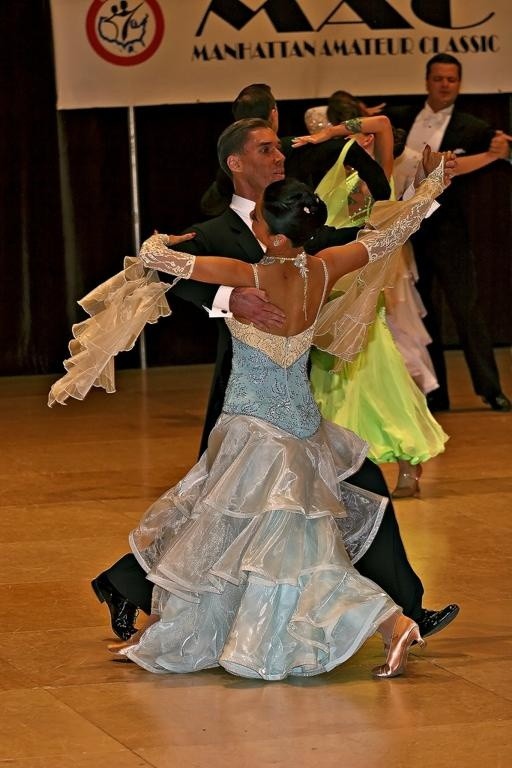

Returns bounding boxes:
[197,85,451,502]
[42,150,459,678]
[306,54,512,412]
[90,115,459,645]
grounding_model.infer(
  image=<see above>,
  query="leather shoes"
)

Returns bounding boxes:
[409,604,460,651]
[90,572,139,641]
[479,391,510,411]
[426,396,451,414]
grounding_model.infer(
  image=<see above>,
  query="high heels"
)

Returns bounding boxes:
[372,615,427,679]
[106,615,162,655]
[391,464,422,498]
